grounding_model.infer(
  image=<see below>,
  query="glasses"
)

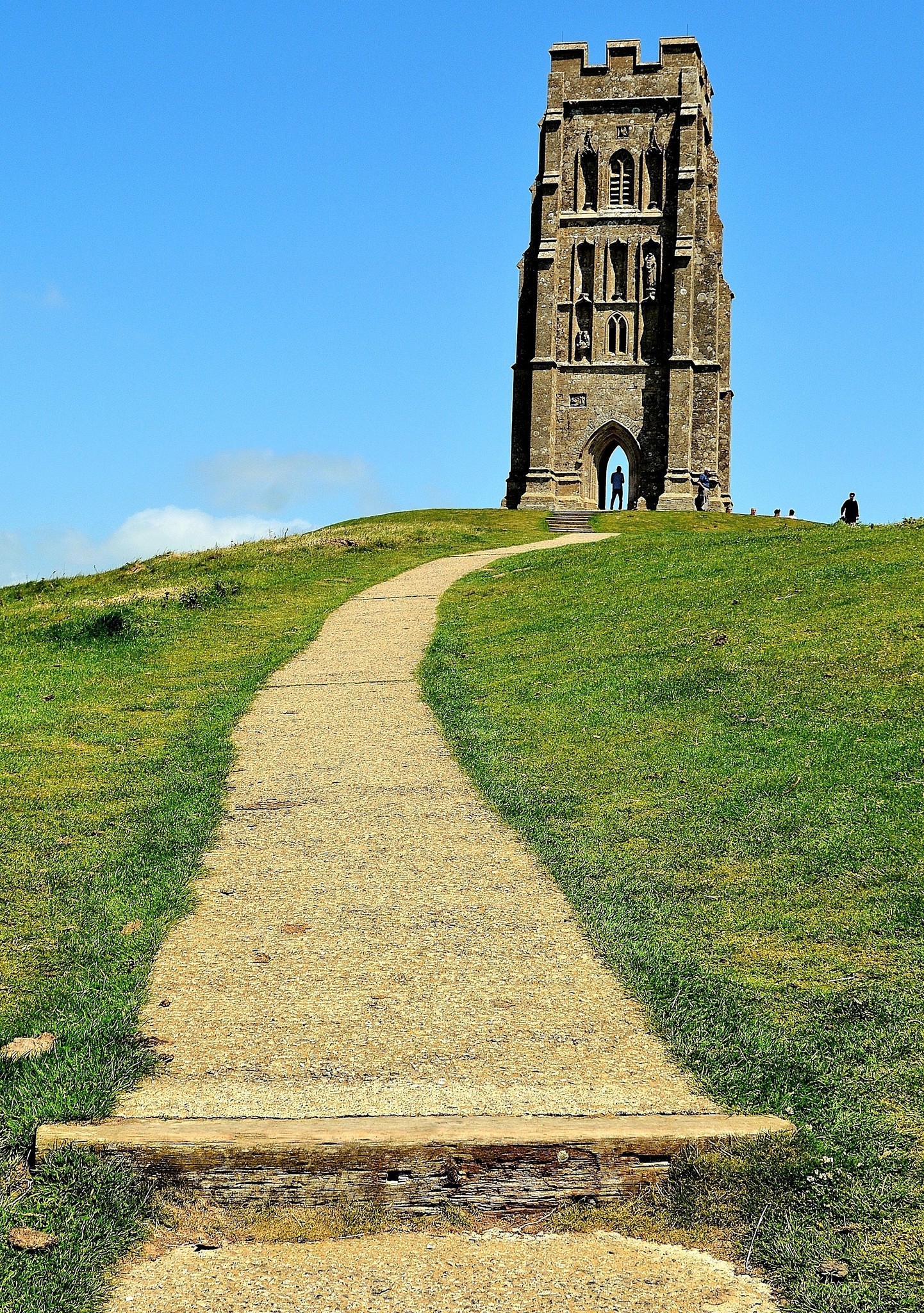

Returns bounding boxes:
[705,470,710,472]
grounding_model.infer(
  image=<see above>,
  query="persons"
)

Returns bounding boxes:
[774,509,781,517]
[726,507,732,513]
[750,508,756,515]
[610,466,624,510]
[697,467,711,511]
[784,510,798,519]
[841,493,859,525]
